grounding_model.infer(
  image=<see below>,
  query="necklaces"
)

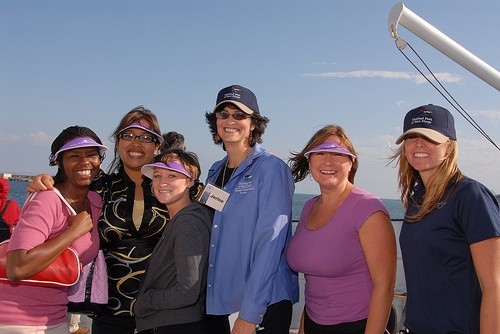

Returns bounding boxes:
[62,183,80,214]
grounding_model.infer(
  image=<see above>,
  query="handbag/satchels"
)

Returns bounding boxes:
[0,187,81,288]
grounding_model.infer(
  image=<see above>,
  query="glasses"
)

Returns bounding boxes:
[118,131,157,143]
[215,109,253,120]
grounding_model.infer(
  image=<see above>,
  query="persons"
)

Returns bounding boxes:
[204,84,300,334]
[0,104,215,334]
[378,103,500,334]
[285,125,397,334]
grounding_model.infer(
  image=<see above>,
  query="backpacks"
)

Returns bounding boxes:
[0,199,11,240]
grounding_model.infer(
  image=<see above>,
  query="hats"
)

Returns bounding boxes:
[396,104,457,144]
[141,161,193,181]
[117,123,164,144]
[49,137,108,161]
[215,85,259,114]
[304,142,356,161]
